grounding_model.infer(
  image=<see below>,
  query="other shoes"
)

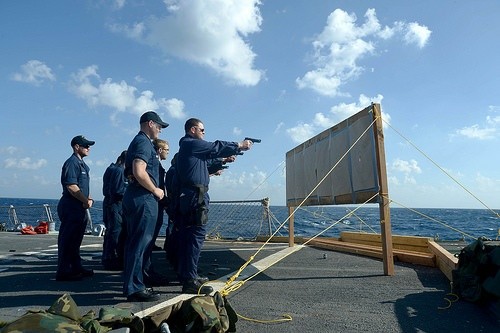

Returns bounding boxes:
[77,267,93,276]
[57,270,84,280]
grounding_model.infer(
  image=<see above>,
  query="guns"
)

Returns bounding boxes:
[219,166,230,169]
[244,137,261,144]
[230,151,244,157]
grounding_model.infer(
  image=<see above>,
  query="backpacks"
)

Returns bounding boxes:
[166,291,238,332]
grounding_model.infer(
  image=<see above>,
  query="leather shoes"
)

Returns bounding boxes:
[127,289,161,301]
[182,280,214,294]
[197,275,210,282]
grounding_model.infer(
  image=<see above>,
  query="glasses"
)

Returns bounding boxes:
[161,148,170,151]
[77,144,90,148]
[193,126,204,132]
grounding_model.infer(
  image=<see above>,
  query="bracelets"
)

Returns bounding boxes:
[89,198,94,203]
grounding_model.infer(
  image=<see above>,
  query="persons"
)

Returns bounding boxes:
[56,135,95,282]
[102,118,253,294]
[122,111,170,301]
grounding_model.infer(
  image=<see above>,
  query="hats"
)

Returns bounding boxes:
[139,111,169,129]
[70,135,95,149]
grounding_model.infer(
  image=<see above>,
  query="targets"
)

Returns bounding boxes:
[285,110,382,205]
